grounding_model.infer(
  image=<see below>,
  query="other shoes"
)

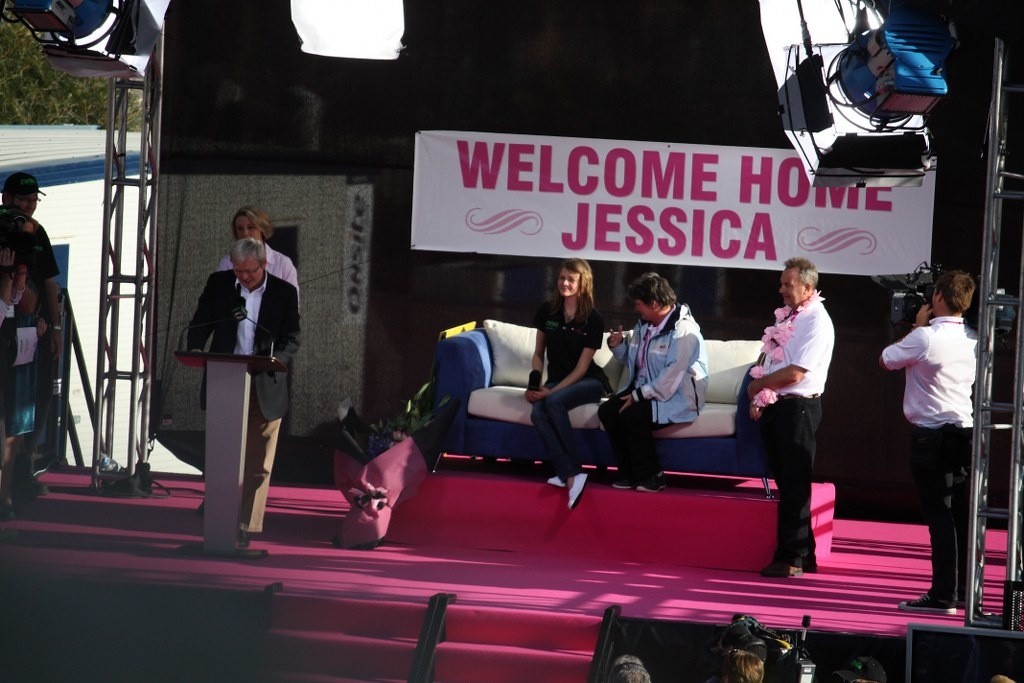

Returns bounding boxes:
[568,473,588,508]
[548,476,566,487]
[33,481,49,495]
[801,549,818,572]
[759,553,803,577]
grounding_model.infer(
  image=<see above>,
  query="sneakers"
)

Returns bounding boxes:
[637,472,667,492]
[612,475,641,489]
[899,593,956,615]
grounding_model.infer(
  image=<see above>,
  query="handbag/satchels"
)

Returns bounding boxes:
[103,460,171,498]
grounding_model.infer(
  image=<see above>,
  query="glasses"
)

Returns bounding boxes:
[10,195,40,204]
[234,263,261,274]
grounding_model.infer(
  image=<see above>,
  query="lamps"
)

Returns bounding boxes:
[776,0,959,188]
[0,0,172,78]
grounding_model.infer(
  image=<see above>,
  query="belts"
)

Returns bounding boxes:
[777,393,818,400]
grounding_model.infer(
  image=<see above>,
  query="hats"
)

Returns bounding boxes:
[3,171,47,195]
[834,656,887,683]
[0,203,27,221]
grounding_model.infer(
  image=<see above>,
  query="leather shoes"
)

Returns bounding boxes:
[239,529,249,547]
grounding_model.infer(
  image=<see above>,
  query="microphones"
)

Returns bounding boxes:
[178,296,275,356]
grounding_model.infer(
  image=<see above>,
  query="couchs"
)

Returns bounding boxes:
[428,320,770,498]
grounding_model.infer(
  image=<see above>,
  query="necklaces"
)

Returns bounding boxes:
[749,289,827,408]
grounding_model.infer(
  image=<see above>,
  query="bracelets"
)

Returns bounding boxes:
[50,324,64,331]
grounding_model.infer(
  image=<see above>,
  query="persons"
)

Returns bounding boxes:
[188,237,301,547]
[526,257,615,509]
[216,205,301,316]
[879,271,981,616]
[0,173,64,521]
[749,257,836,578]
[608,648,1018,683]
[597,270,710,492]
